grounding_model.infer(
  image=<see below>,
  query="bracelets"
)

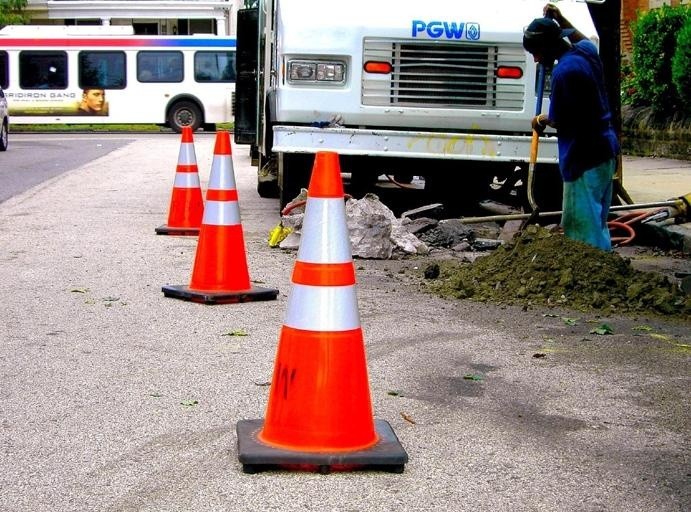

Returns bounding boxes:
[536,114,548,129]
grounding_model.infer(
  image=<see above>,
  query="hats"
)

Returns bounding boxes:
[528,18,577,39]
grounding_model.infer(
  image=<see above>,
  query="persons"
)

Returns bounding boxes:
[73,90,108,116]
[522,2,622,252]
[139,58,237,81]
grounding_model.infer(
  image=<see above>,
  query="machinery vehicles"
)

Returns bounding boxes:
[235,0,603,228]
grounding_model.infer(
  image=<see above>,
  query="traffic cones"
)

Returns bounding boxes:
[163,128,280,306]
[240,153,409,474]
[154,125,203,236]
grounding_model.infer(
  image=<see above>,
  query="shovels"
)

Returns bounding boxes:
[521,4,556,228]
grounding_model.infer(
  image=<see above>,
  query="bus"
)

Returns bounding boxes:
[1,22,236,135]
